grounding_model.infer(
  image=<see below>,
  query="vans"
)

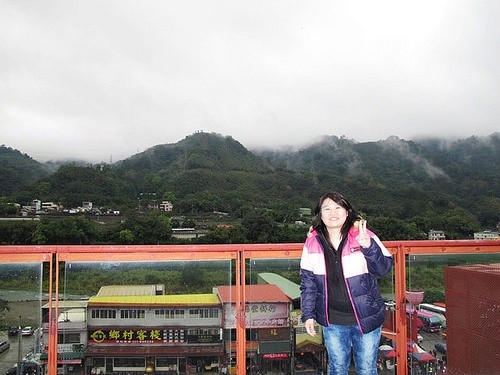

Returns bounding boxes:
[5,367,33,375]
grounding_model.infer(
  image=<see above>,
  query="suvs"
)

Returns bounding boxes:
[0,342,11,355]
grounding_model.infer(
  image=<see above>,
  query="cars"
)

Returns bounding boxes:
[34,328,43,338]
[22,326,35,335]
[8,327,18,336]
[418,335,423,342]
[435,342,446,353]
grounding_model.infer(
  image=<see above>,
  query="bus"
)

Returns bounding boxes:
[406,305,442,333]
[443,318,447,338]
[418,302,446,317]
